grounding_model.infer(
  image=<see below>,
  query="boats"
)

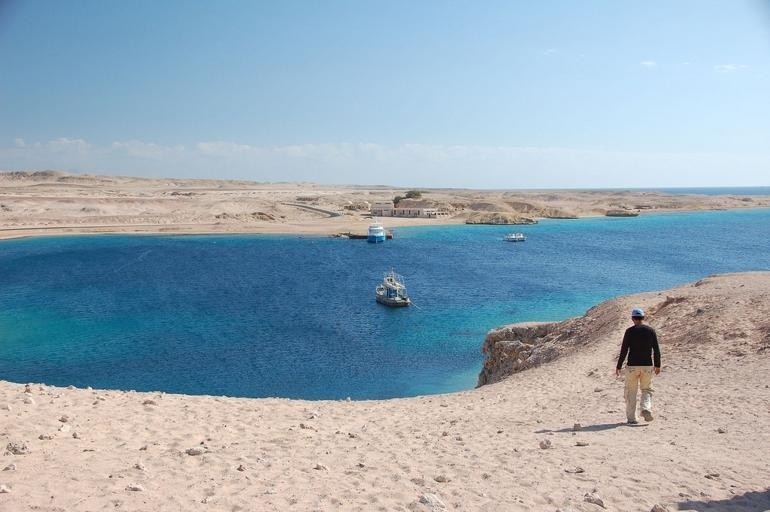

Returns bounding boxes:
[368,225,386,244]
[503,233,527,241]
[375,268,410,307]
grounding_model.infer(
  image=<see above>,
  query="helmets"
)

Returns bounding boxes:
[631,308,645,317]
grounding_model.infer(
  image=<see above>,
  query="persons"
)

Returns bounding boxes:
[616,308,661,423]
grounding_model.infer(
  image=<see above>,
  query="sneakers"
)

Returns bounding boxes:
[627,420,638,424]
[641,410,653,422]
